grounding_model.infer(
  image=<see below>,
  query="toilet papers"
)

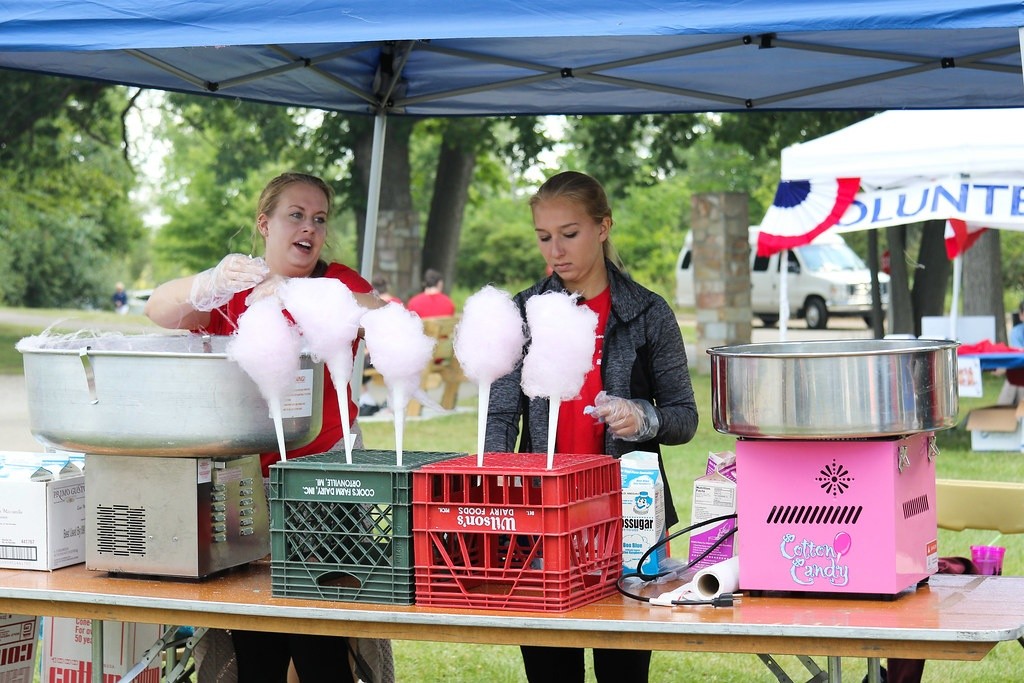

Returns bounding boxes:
[692,556,739,600]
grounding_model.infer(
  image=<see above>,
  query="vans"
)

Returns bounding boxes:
[674,227,888,331]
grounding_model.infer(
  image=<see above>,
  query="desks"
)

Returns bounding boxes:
[0,561,1024,683]
[920,316,1024,371]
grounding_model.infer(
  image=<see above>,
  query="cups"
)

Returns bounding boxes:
[970,545,1006,576]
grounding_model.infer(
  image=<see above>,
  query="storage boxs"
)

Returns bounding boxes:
[964,400,1024,452]
[0,447,84,573]
[410,452,622,612]
[267,450,469,606]
[0,613,40,683]
[41,616,162,683]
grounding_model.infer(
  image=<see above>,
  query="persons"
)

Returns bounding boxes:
[407,270,455,364]
[361,276,406,384]
[112,283,129,314]
[144,174,387,683]
[1005,302,1024,386]
[484,170,699,683]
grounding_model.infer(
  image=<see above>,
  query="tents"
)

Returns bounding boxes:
[0,0,1024,440]
[757,109,1024,341]
